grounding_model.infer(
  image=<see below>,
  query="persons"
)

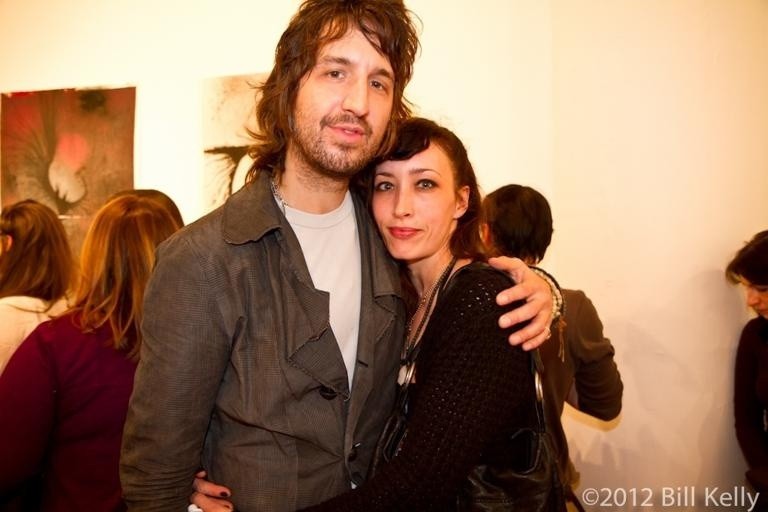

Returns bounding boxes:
[0,1,622,511]
[725,230,767,512]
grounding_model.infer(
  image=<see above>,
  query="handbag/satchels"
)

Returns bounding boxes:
[457,428,568,512]
